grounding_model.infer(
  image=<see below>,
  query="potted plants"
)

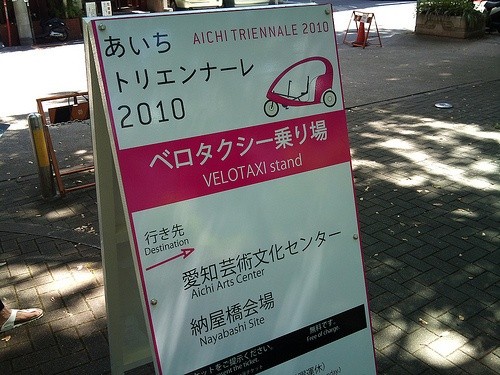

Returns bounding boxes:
[416,0,486,38]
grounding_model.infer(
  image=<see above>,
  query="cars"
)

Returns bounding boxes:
[167,0,223,11]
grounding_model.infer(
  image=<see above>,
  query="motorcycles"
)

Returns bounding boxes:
[32,13,69,42]
[483,0,500,34]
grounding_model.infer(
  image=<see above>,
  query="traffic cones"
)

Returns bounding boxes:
[352,22,368,45]
